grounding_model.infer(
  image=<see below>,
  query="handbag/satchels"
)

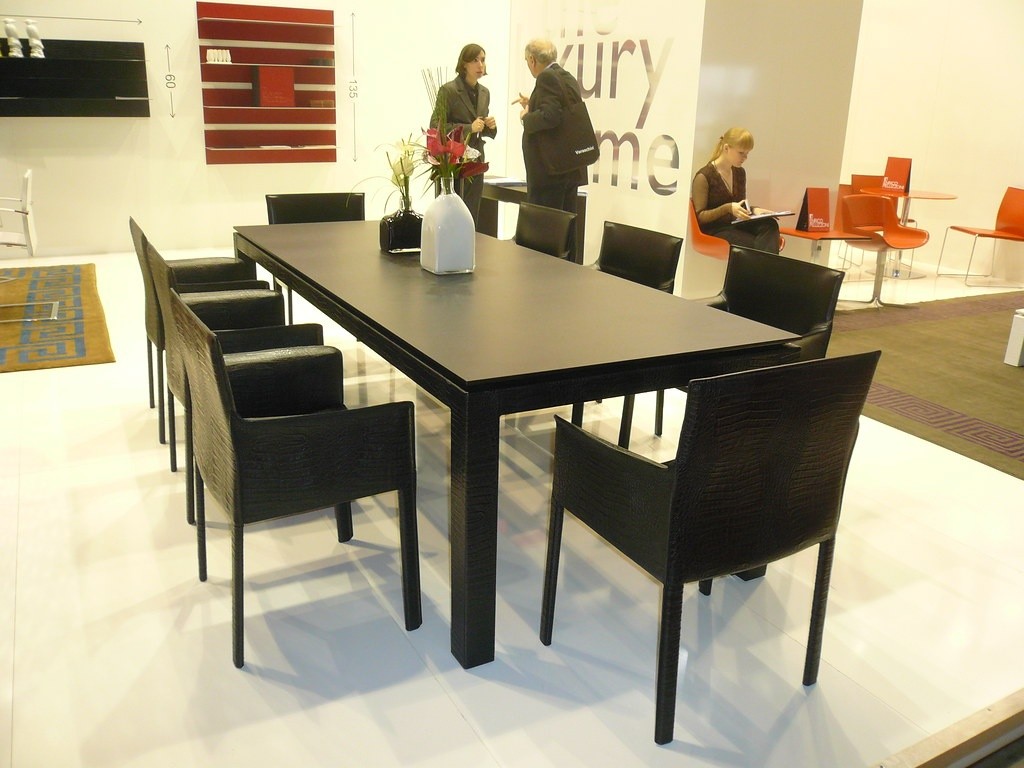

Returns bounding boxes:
[534,69,600,175]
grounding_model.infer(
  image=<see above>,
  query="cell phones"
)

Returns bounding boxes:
[741,201,750,213]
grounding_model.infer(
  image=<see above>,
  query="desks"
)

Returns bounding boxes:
[858,186,957,280]
[476,175,585,264]
[780,224,873,266]
[233,221,801,673]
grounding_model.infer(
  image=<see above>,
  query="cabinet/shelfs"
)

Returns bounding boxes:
[196,0,338,165]
[0,37,151,118]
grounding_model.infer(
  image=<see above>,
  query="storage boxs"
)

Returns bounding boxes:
[310,99,323,107]
[323,100,334,107]
[251,64,295,108]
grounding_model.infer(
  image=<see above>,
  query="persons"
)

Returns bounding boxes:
[693,125,780,257]
[511,40,600,263]
[429,44,497,232]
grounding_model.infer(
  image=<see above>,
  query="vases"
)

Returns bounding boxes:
[418,175,477,276]
[378,196,424,253]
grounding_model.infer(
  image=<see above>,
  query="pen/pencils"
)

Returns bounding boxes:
[744,200,752,216]
[475,133,479,139]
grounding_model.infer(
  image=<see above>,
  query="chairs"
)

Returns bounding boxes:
[936,185,1024,289]
[0,169,38,257]
[585,219,685,447]
[145,240,325,473]
[265,192,367,226]
[654,244,845,437]
[129,215,270,445]
[851,173,918,283]
[840,193,929,310]
[834,184,865,271]
[688,197,786,261]
[536,348,885,749]
[168,285,422,669]
[505,202,578,262]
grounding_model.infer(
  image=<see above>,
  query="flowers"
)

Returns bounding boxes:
[341,129,439,212]
[394,65,489,195]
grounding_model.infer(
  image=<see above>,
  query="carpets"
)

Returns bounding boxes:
[824,290,1024,482]
[0,263,116,374]
